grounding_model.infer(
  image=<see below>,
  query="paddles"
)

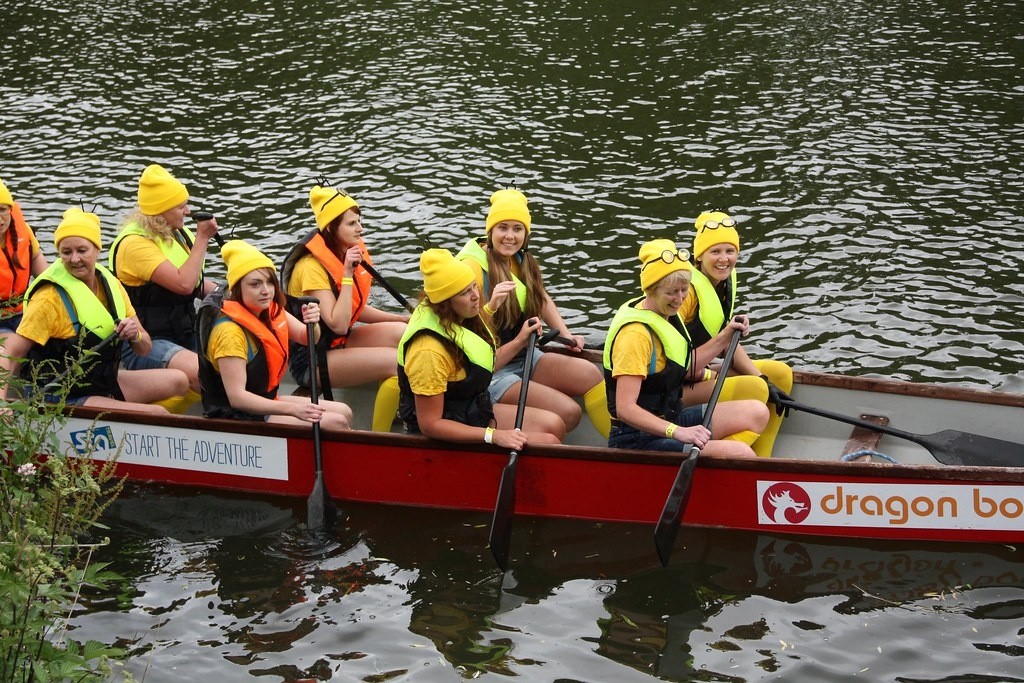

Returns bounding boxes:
[538,327,606,350]
[13,318,122,419]
[297,295,337,532]
[191,211,226,248]
[353,256,416,315]
[485,319,541,575]
[651,314,744,570]
[775,404,1024,467]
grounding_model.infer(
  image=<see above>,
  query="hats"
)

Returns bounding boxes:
[221,240,276,290]
[486,189,530,236]
[694,211,740,260]
[137,164,188,216]
[55,208,102,252]
[638,239,689,289]
[310,185,359,232]
[0,178,12,206]
[420,248,475,303]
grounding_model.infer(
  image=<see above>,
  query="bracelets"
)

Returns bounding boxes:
[342,278,353,285]
[130,330,142,343]
[483,303,497,316]
[697,368,711,382]
[666,423,678,439]
[484,427,495,444]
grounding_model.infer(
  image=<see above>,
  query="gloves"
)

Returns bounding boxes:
[760,376,796,418]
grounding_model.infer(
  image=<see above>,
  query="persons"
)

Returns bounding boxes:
[390,249,566,451]
[675,212,797,457]
[109,164,218,414]
[0,208,190,423]
[455,190,611,438]
[602,239,770,461]
[0,177,48,351]
[280,185,411,432]
[195,240,354,431]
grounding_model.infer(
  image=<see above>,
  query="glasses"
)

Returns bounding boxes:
[320,187,348,209]
[642,249,690,271]
[701,217,737,234]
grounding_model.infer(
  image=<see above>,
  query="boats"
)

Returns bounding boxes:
[1,345,1024,544]
